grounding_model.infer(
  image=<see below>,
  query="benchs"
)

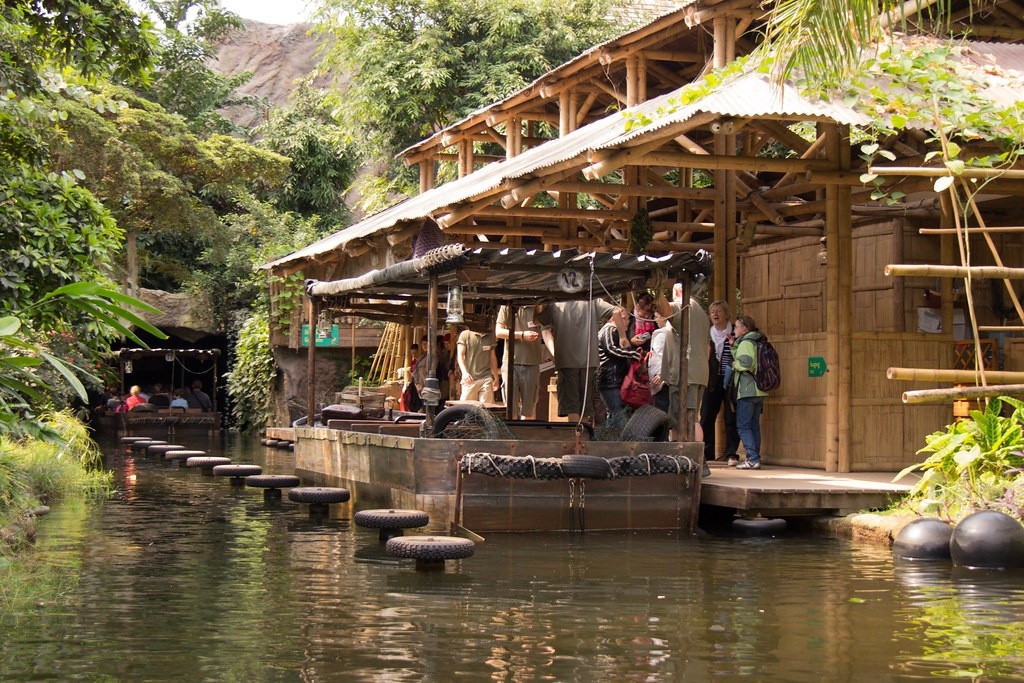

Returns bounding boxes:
[158,409,201,414]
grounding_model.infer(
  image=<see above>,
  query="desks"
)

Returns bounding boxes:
[335,390,386,409]
[444,400,507,421]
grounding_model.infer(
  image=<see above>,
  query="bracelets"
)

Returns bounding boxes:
[521,330,524,341]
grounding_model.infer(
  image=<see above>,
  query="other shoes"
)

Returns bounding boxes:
[736,458,761,469]
[727,456,738,466]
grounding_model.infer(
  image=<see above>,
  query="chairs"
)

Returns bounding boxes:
[130,403,157,413]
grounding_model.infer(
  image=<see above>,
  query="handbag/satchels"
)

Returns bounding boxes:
[402,382,422,412]
[620,348,652,407]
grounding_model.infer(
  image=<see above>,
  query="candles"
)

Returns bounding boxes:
[359,377,363,396]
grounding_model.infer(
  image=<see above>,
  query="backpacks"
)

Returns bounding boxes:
[741,339,781,392]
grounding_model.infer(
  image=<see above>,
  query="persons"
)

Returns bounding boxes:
[534,297,631,423]
[652,277,711,477]
[597,292,672,426]
[700,299,740,466]
[726,315,769,469]
[95,381,212,414]
[457,329,499,403]
[406,333,462,415]
[496,301,555,420]
